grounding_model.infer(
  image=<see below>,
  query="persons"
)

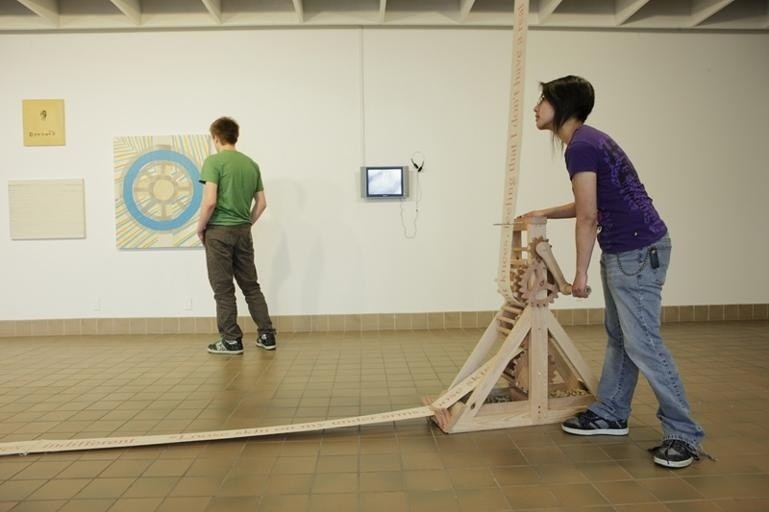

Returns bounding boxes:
[196,118,278,354]
[513,73,718,470]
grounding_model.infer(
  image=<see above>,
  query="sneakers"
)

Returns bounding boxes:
[652,433,700,467]
[207,335,244,355]
[560,408,631,436]
[255,333,278,350]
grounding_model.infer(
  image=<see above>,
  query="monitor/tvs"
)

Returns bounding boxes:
[360,165,409,201]
